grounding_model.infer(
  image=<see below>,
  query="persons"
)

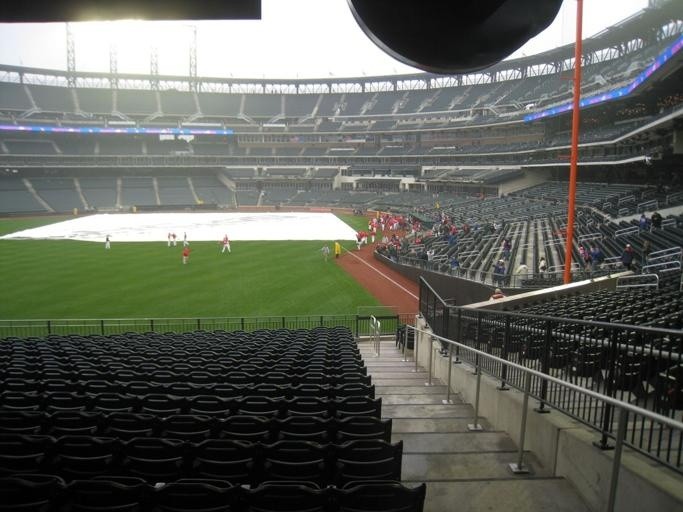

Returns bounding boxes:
[489,288,503,301]
[641,240,655,274]
[167,232,190,266]
[513,262,529,284]
[538,257,548,280]
[221,234,232,255]
[620,243,634,270]
[640,212,666,232]
[576,242,607,275]
[105,235,111,250]
[319,191,511,286]
